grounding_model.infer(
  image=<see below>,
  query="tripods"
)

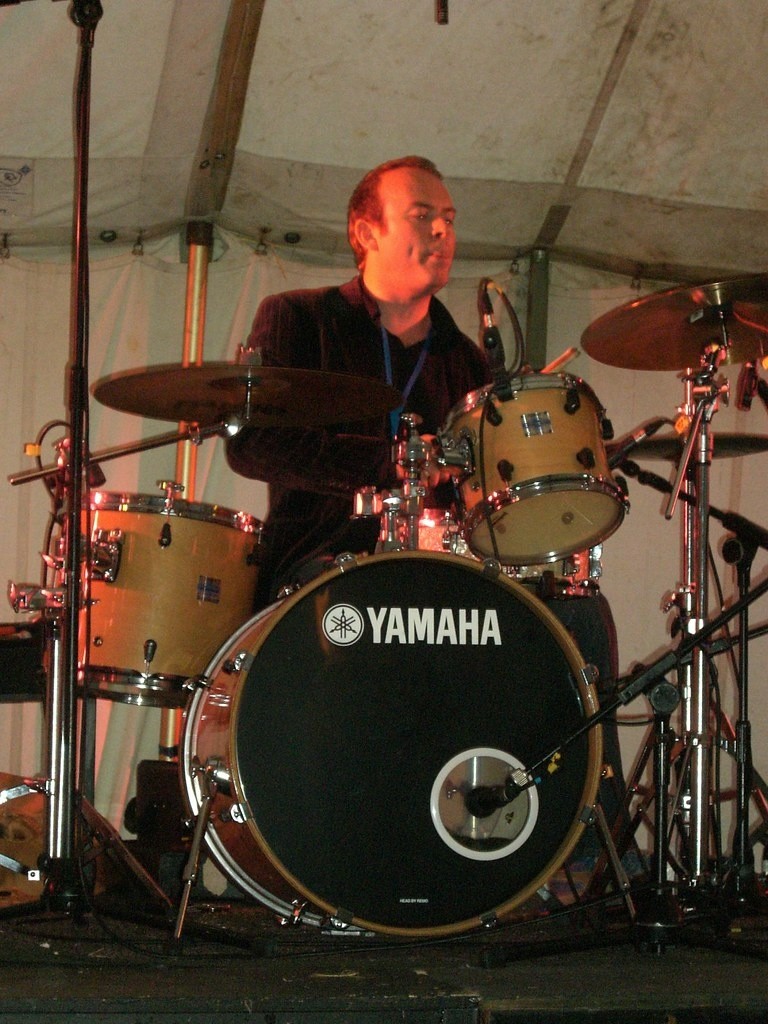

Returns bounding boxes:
[0,615,180,924]
[477,344,768,973]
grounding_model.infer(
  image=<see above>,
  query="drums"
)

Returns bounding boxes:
[50,488,271,711]
[172,550,607,936]
[447,373,631,567]
[0,622,48,703]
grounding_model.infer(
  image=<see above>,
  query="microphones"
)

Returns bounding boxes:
[465,768,530,818]
[87,461,107,488]
[475,277,505,367]
[737,359,759,412]
[607,418,667,471]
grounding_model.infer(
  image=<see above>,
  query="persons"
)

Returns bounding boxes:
[226,156,491,623]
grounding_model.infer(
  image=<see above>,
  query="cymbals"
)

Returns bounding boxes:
[579,268,767,371]
[89,362,402,441]
[610,431,768,460]
